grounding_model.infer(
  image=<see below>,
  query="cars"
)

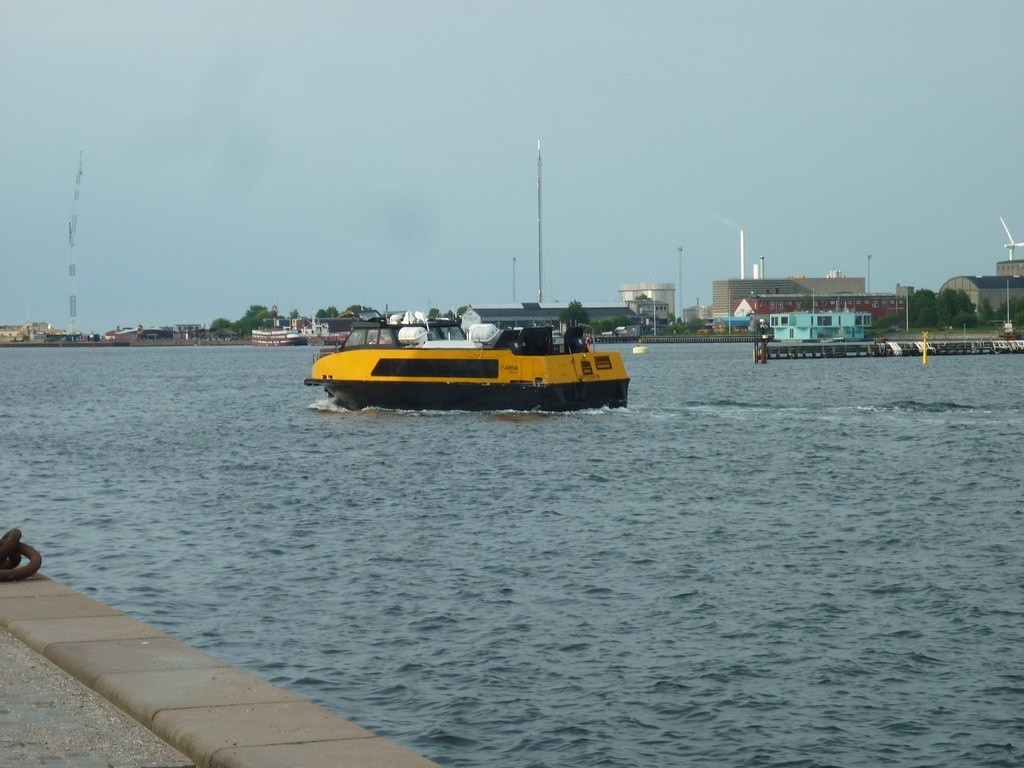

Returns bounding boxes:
[887,326,902,333]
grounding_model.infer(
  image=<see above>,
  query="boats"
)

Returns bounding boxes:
[303,304,630,412]
[250,326,301,345]
